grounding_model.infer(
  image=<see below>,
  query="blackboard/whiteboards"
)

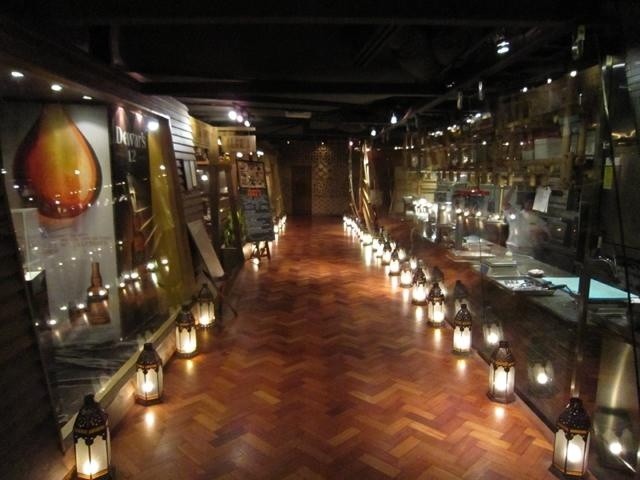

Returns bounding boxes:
[186,217,225,280]
[235,159,275,242]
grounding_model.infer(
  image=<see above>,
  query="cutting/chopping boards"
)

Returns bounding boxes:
[541,276,639,303]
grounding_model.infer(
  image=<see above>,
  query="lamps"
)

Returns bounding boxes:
[175,304,198,358]
[373,232,382,251]
[250,212,286,264]
[528,337,560,398]
[396,243,408,263]
[399,261,411,289]
[415,259,430,282]
[381,243,392,265]
[548,398,592,480]
[411,269,426,305]
[343,214,372,246]
[135,343,165,406]
[450,304,475,355]
[407,252,418,271]
[197,282,215,328]
[485,339,517,404]
[425,282,446,326]
[375,233,386,259]
[72,392,114,480]
[431,266,448,299]
[388,249,401,277]
[380,225,396,251]
[480,308,507,350]
[452,279,474,319]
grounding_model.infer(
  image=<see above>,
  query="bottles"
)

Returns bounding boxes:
[85,261,110,325]
